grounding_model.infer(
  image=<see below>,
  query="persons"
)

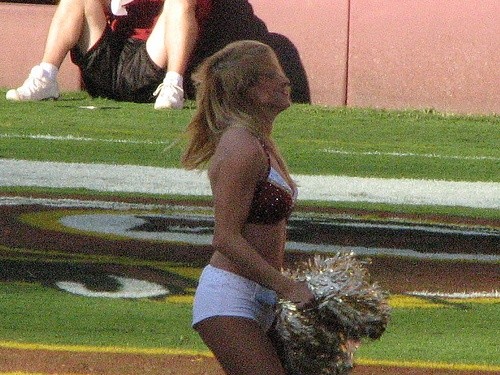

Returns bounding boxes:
[183,40,313,375]
[6,0,211,110]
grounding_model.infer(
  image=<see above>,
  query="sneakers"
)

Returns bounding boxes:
[6,65,59,101]
[153,77,185,110]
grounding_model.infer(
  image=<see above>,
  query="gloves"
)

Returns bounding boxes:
[111,0,133,16]
